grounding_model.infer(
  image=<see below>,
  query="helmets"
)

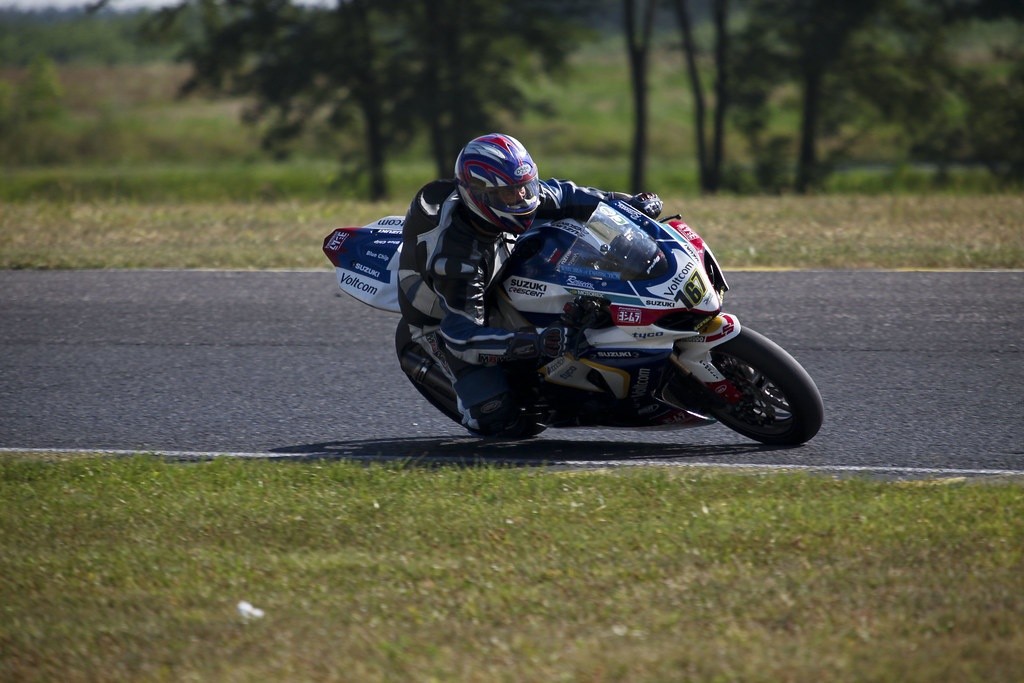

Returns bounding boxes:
[455,133,541,235]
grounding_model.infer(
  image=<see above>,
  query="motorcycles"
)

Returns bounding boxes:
[323,215,824,449]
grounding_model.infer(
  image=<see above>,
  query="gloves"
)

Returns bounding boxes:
[610,191,663,219]
[501,324,572,359]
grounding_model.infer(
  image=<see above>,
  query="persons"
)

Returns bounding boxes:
[398,134,662,437]
